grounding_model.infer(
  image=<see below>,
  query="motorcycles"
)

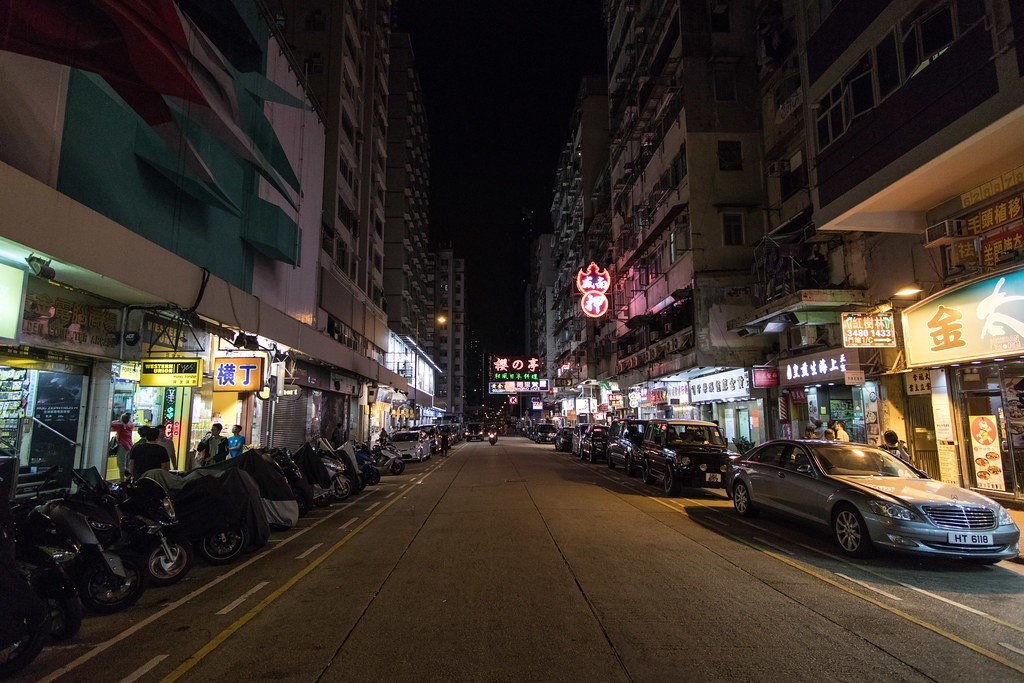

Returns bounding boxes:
[0,437,407,678]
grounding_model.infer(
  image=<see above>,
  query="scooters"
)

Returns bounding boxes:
[488,432,498,446]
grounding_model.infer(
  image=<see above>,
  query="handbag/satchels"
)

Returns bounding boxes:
[109,436,119,456]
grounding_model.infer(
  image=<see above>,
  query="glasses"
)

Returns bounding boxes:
[211,428,217,431]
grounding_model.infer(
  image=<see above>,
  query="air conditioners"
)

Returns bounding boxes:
[767,162,790,177]
[924,219,963,241]
[618,324,686,372]
[623,162,668,194]
[788,324,819,348]
[616,2,679,93]
[631,259,645,271]
[803,222,830,242]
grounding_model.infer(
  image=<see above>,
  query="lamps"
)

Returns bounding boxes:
[24,257,57,280]
[897,285,923,296]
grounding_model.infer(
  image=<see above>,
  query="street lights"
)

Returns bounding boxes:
[438,316,446,423]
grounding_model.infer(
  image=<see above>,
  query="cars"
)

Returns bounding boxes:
[389,432,433,463]
[521,423,557,444]
[554,427,574,452]
[606,418,667,477]
[410,423,465,455]
[465,422,485,442]
[640,419,742,497]
[725,438,1021,565]
[572,423,591,457]
[578,424,610,464]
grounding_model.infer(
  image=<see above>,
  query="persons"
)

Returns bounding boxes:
[379,428,388,439]
[877,430,911,462]
[439,426,449,457]
[155,425,177,470]
[803,426,817,438]
[332,423,342,448]
[836,421,849,441]
[227,425,245,457]
[824,429,838,441]
[824,420,837,439]
[126,425,150,480]
[112,412,136,483]
[128,428,170,483]
[488,425,497,435]
[197,423,229,467]
[812,420,827,440]
